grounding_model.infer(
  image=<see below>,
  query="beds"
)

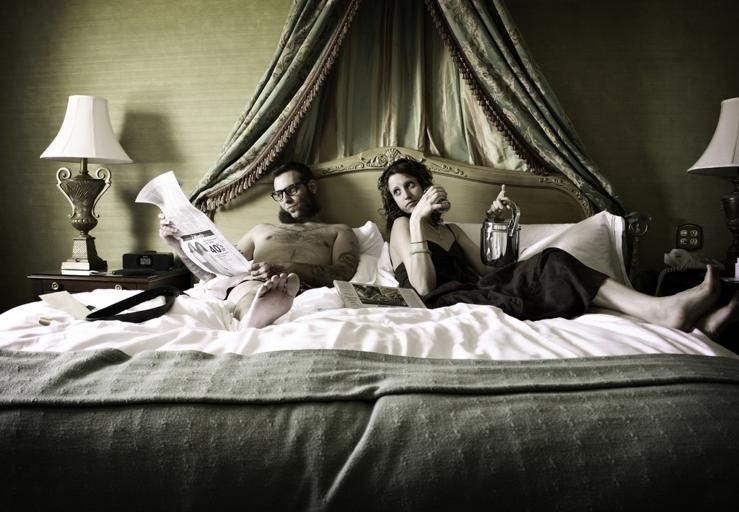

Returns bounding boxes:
[2,146,737,506]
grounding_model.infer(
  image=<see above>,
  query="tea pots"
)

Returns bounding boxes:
[480,199,521,267]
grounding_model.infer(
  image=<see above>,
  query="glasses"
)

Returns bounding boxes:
[271,181,303,202]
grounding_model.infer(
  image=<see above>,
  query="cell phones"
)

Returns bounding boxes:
[113,270,155,275]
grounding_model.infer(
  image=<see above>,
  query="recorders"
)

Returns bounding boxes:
[122,252,174,269]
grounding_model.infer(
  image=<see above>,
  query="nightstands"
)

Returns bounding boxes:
[26,271,191,299]
[647,268,707,296]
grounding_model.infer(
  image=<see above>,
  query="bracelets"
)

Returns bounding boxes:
[409,238,429,246]
[410,249,433,257]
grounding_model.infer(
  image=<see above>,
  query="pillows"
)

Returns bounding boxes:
[516,209,634,290]
[350,219,386,287]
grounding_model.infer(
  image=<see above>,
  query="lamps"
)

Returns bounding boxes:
[37,94,133,276]
[688,97,738,275]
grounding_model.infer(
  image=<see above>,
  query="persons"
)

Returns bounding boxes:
[157,160,360,331]
[374,158,738,341]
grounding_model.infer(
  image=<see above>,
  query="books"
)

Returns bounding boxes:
[330,278,427,311]
